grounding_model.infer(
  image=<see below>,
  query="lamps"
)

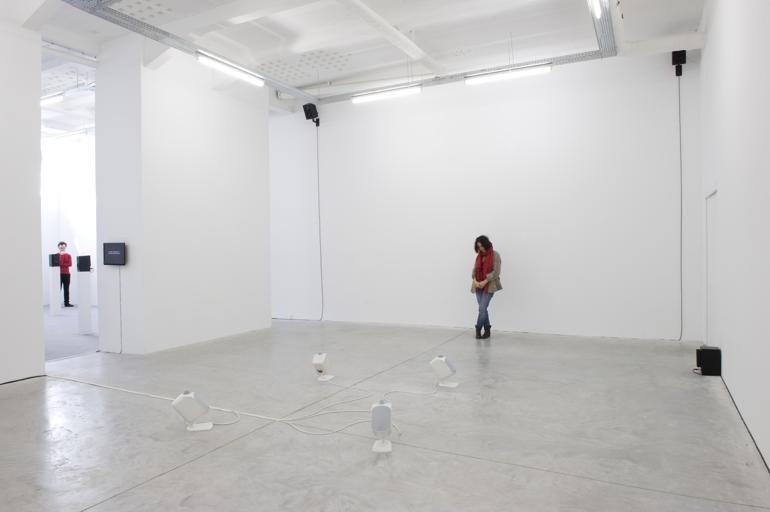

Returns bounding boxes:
[169,387,214,434]
[461,61,552,85]
[191,49,266,90]
[346,83,425,106]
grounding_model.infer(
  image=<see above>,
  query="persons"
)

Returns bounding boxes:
[58,242,74,307]
[471,235,502,339]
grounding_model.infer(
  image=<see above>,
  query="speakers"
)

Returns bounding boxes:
[171,390,213,432]
[303,103,319,120]
[672,50,686,65]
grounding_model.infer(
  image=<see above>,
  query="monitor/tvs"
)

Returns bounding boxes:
[77,256,90,271]
[103,242,126,266]
[49,254,59,266]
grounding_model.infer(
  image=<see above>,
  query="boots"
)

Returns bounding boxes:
[481,326,491,339]
[475,325,482,339]
